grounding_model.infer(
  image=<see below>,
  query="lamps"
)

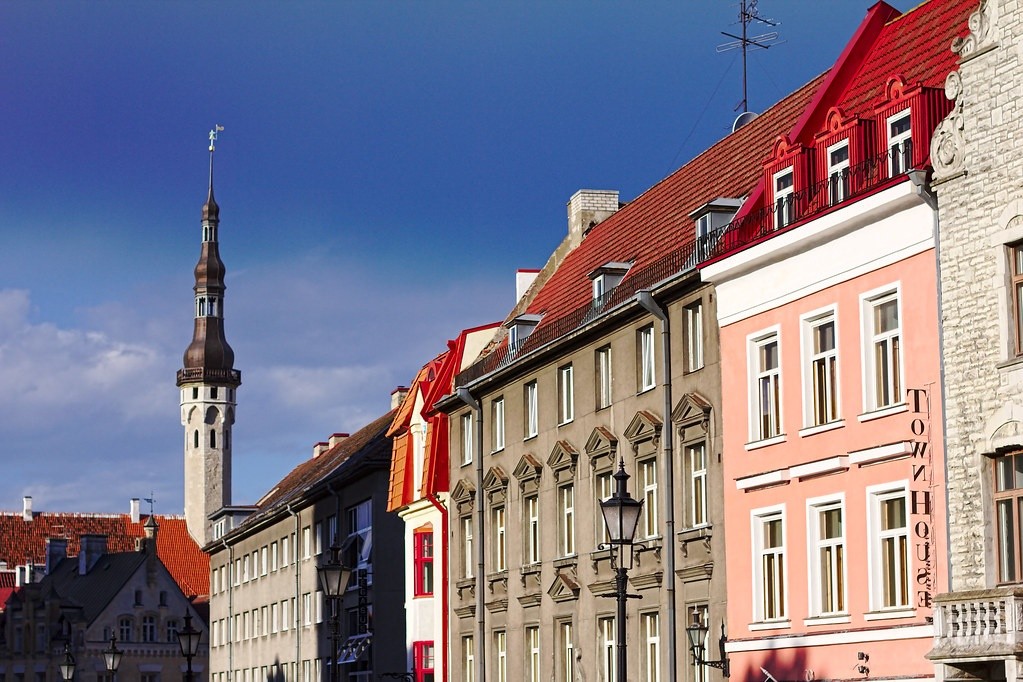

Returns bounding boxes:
[685,603,730,679]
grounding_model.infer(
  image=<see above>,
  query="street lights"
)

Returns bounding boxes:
[102,629,124,682]
[598,457,646,682]
[174,607,203,682]
[314,533,354,682]
